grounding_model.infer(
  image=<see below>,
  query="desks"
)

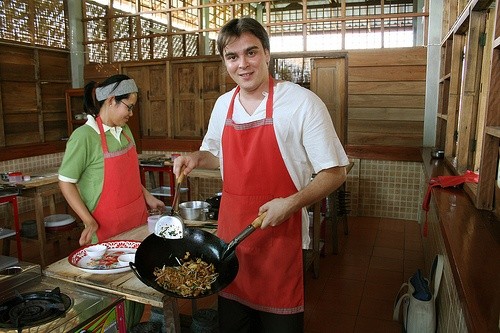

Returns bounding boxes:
[0,152,355,333]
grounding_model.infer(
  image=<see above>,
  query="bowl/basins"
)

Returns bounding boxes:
[117,254,135,266]
[430,159,442,166]
[8,172,22,182]
[171,154,181,161]
[0,171,13,180]
[147,214,162,233]
[85,245,107,260]
[431,151,444,159]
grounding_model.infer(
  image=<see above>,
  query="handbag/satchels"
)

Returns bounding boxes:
[392,254,444,333]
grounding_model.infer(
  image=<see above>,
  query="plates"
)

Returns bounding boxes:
[43,213,76,228]
[45,222,76,232]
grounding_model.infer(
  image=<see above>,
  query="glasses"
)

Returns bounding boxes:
[116,98,134,112]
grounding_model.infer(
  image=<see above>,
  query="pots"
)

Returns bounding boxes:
[129,210,267,299]
[179,200,214,221]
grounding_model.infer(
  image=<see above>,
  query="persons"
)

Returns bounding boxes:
[58,74,165,331]
[174,18,349,333]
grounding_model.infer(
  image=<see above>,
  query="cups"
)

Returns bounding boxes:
[22,220,37,238]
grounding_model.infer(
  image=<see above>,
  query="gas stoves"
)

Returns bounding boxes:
[0,275,126,333]
